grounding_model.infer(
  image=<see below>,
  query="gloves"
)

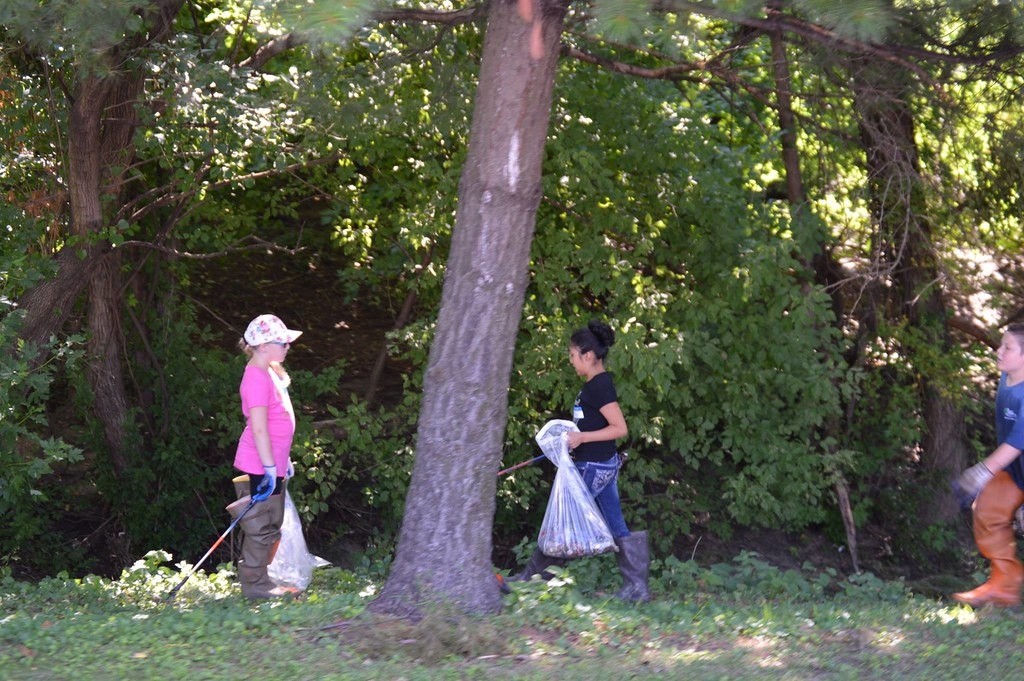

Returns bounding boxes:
[287,457,295,480]
[951,461,993,509]
[252,466,277,501]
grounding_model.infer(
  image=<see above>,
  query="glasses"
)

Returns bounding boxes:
[269,342,287,348]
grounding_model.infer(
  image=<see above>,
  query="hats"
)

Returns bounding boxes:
[244,314,303,345]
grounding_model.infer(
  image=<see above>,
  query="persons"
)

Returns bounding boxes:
[227,314,303,600]
[950,323,1024,608]
[496,323,649,603]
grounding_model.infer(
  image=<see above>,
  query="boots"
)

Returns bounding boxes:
[494,545,564,594]
[952,469,1023,609]
[227,475,306,601]
[612,530,651,603]
